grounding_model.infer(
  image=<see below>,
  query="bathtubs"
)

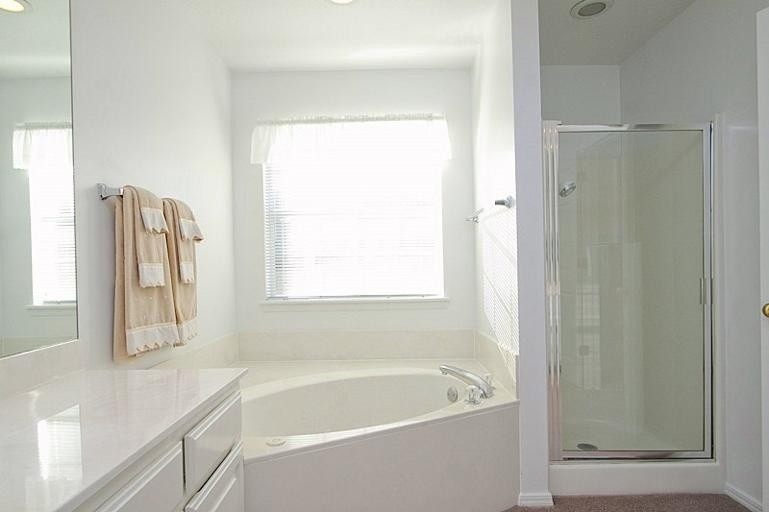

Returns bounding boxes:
[240,359,519,510]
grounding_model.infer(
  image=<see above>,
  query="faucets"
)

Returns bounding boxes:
[441,366,492,398]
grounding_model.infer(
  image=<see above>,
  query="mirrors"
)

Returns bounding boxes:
[0,0,88,361]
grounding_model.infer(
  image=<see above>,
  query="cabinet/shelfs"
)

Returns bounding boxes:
[0,362,254,512]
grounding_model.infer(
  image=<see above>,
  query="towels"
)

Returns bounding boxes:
[165,193,206,348]
[111,185,176,365]
